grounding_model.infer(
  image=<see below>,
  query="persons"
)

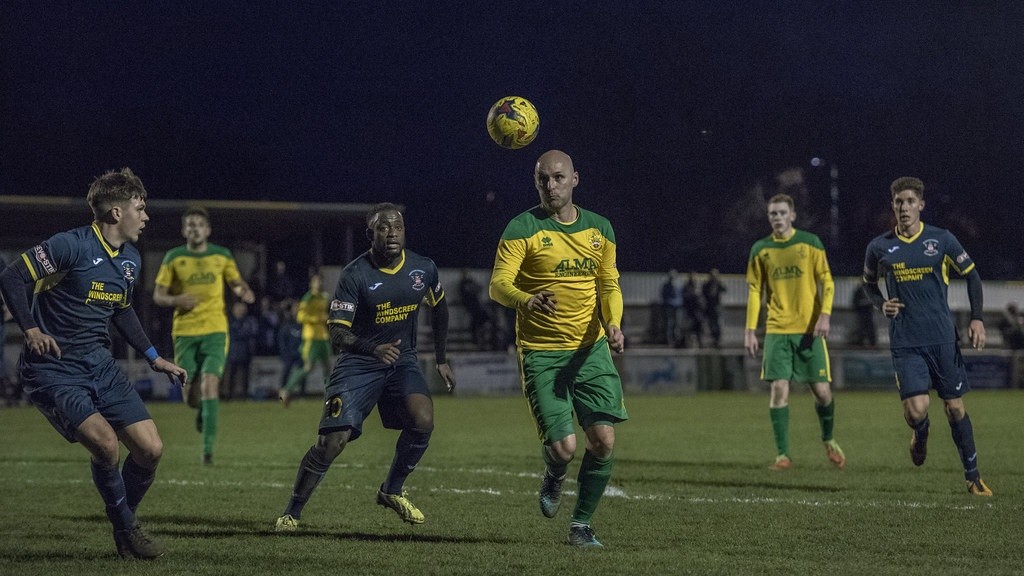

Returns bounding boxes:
[459,270,518,352]
[275,203,456,532]
[853,284,875,346]
[1,173,188,560]
[659,268,727,348]
[862,177,993,496]
[227,298,307,401]
[998,303,1024,348]
[279,272,333,404]
[153,207,254,464]
[744,194,845,468]
[489,150,628,546]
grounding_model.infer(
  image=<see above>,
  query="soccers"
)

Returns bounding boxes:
[487,96,540,150]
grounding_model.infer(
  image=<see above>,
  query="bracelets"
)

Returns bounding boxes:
[143,347,158,361]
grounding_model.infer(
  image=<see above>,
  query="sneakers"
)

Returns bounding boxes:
[825,438,845,468]
[375,482,425,525]
[568,526,603,548]
[768,453,790,470]
[118,519,162,558]
[274,515,299,532]
[539,467,568,519]
[116,540,136,561]
[965,477,993,497]
[910,427,930,466]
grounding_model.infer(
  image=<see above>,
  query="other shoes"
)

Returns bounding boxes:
[279,388,290,406]
[203,452,213,466]
[195,409,204,433]
